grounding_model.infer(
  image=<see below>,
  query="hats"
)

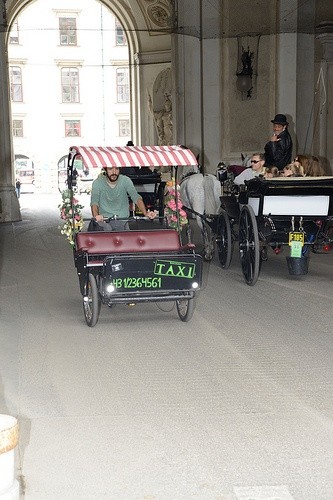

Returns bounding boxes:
[271,114,289,125]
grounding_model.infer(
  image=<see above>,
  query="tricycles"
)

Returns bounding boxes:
[65,144,205,327]
[114,171,167,224]
[75,178,94,195]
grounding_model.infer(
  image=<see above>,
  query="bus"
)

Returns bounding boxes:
[17,169,72,185]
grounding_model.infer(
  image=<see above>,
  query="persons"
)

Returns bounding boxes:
[232,114,293,186]
[90,167,156,231]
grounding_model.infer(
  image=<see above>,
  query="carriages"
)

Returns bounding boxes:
[177,153,333,286]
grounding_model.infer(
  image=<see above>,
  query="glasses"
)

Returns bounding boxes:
[251,160,259,163]
[294,159,298,162]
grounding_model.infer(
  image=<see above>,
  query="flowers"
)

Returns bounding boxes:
[165,179,188,232]
[58,189,83,245]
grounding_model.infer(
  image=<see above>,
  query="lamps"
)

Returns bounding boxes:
[236,52,253,97]
